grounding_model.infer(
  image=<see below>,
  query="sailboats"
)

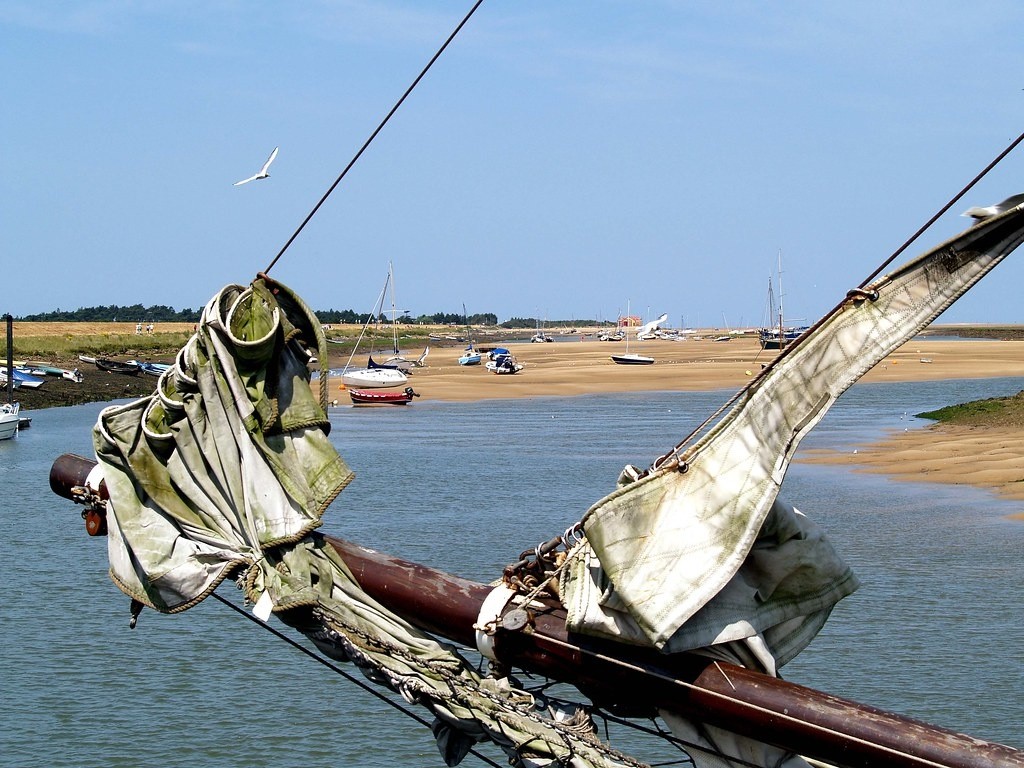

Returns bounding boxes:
[759,275,788,350]
[607,298,654,365]
[758,276,810,345]
[457,301,482,366]
[340,259,408,388]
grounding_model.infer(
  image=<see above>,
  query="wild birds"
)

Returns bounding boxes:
[232,146,279,186]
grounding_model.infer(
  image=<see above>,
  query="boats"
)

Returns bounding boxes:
[79,355,95,363]
[486,347,513,360]
[18,418,32,428]
[0,360,85,389]
[485,360,524,374]
[347,387,420,405]
[531,300,764,343]
[135,360,173,377]
[0,402,20,440]
[381,346,432,368]
[95,360,142,376]
[325,338,344,344]
[427,333,477,343]
